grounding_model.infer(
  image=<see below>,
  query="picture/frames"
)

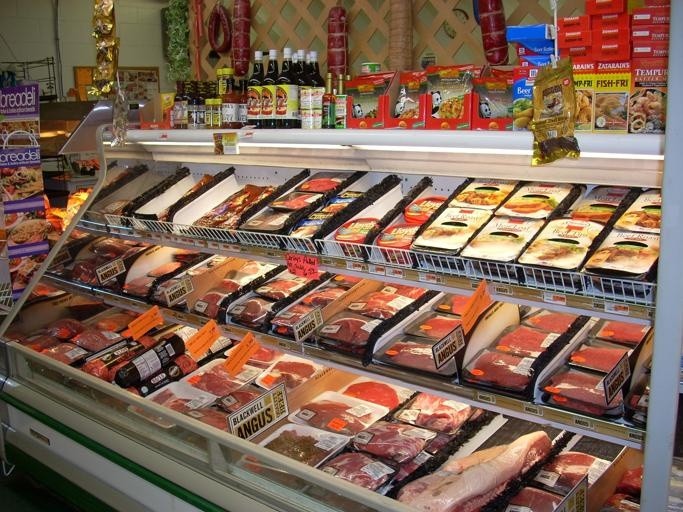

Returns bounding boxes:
[72,64,159,106]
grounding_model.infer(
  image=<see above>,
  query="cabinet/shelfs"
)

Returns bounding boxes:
[41,101,113,192]
[0,122,683,512]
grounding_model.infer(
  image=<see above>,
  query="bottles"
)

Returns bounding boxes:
[246,50,265,127]
[322,72,335,129]
[216,68,223,96]
[237,78,248,127]
[204,98,213,128]
[221,67,234,95]
[262,49,278,127]
[294,50,311,129]
[308,51,324,129]
[276,47,300,128]
[335,74,347,128]
[173,80,207,129]
[212,99,223,129]
[223,78,239,128]
